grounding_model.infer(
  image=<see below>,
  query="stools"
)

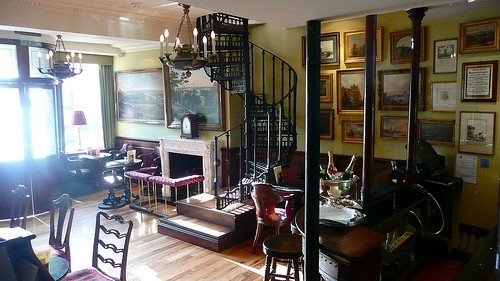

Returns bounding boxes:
[95,169,131,208]
[262,220,302,281]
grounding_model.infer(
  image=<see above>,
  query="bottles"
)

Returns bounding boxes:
[319,151,357,193]
[86,146,100,155]
[127,145,134,150]
[391,161,401,179]
[385,233,392,250]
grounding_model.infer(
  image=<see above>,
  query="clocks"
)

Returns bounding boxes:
[179,113,199,139]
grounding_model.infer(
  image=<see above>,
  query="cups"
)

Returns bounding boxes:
[124,157,129,164]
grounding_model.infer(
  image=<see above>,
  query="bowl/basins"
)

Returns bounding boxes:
[319,174,360,199]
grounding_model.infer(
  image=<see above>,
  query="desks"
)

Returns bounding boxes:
[80,152,111,161]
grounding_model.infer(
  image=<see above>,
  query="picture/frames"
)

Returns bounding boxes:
[320,73,334,139]
[340,119,364,144]
[301,31,340,68]
[162,64,225,131]
[337,68,365,115]
[114,67,165,124]
[458,16,500,155]
[344,27,382,64]
[379,28,456,146]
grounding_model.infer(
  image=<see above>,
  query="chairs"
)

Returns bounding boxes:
[10,185,30,230]
[60,210,133,281]
[251,182,295,247]
[34,194,73,274]
[58,141,161,210]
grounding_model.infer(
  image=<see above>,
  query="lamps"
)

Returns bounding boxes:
[38,35,83,84]
[158,5,216,77]
[70,110,87,151]
